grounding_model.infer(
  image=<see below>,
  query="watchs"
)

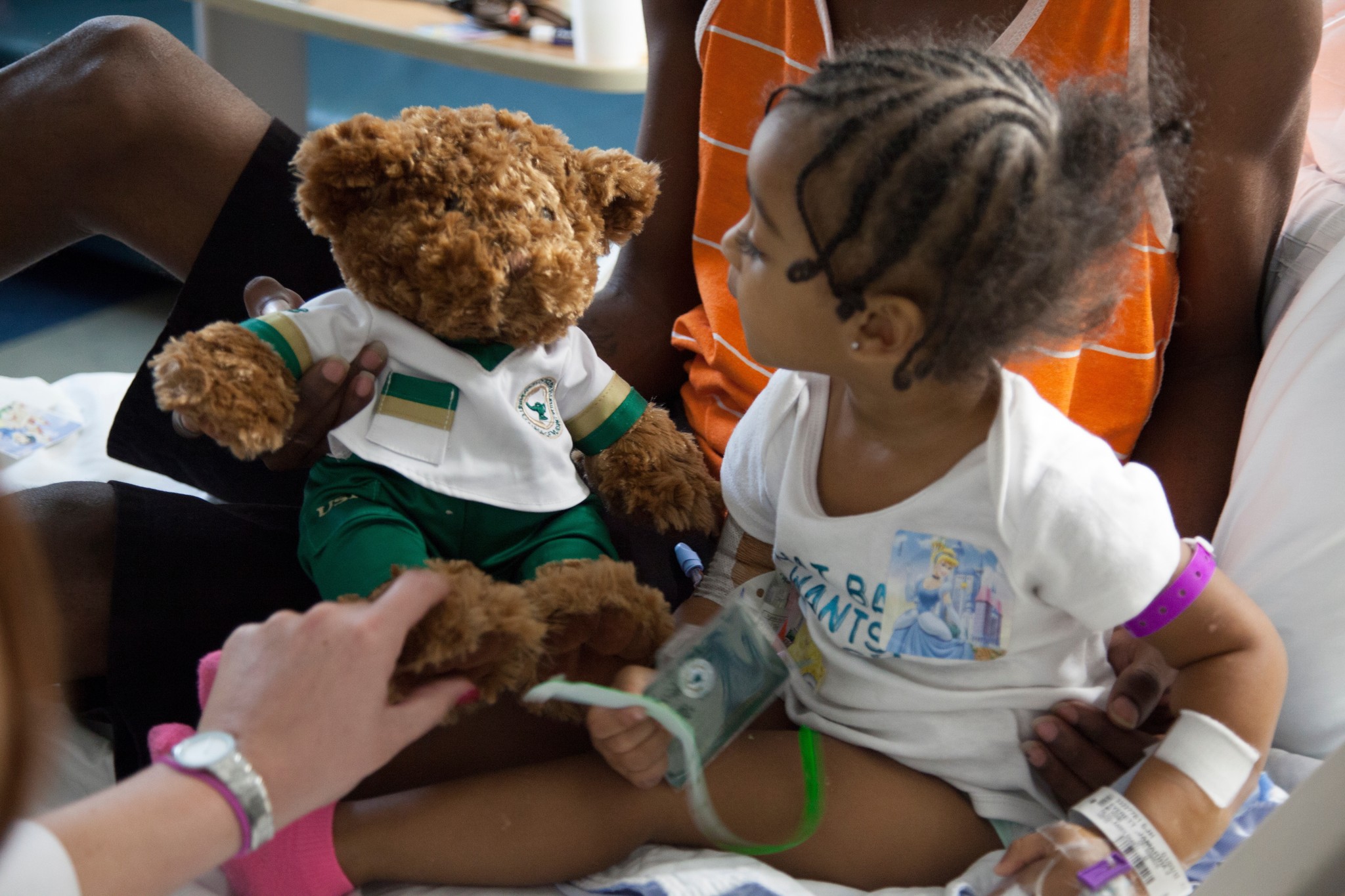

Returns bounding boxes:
[167,728,281,848]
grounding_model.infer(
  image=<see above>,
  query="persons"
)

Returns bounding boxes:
[0,1,1323,816]
[147,37,1290,896]
[0,465,488,896]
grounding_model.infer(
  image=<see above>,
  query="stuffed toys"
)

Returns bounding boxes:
[142,102,721,729]
